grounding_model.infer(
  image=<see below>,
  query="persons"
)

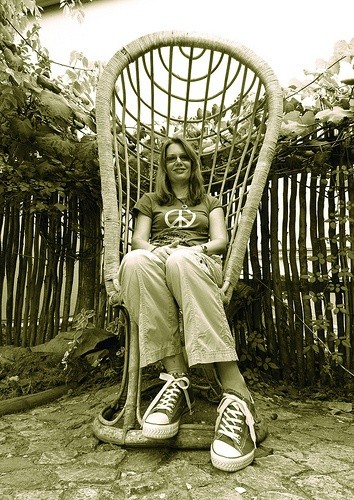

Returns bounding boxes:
[117,136,257,472]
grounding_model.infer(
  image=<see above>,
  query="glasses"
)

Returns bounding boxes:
[166,155,189,162]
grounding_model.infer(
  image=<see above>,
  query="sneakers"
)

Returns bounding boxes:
[210,389,256,472]
[140,372,195,439]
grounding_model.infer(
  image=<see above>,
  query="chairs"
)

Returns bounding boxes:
[96,31,283,448]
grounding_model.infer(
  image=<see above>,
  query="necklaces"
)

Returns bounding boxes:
[176,197,189,210]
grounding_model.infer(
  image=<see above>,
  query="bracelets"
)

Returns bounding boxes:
[150,246,159,252]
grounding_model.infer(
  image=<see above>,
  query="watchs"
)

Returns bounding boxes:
[200,245,208,255]
